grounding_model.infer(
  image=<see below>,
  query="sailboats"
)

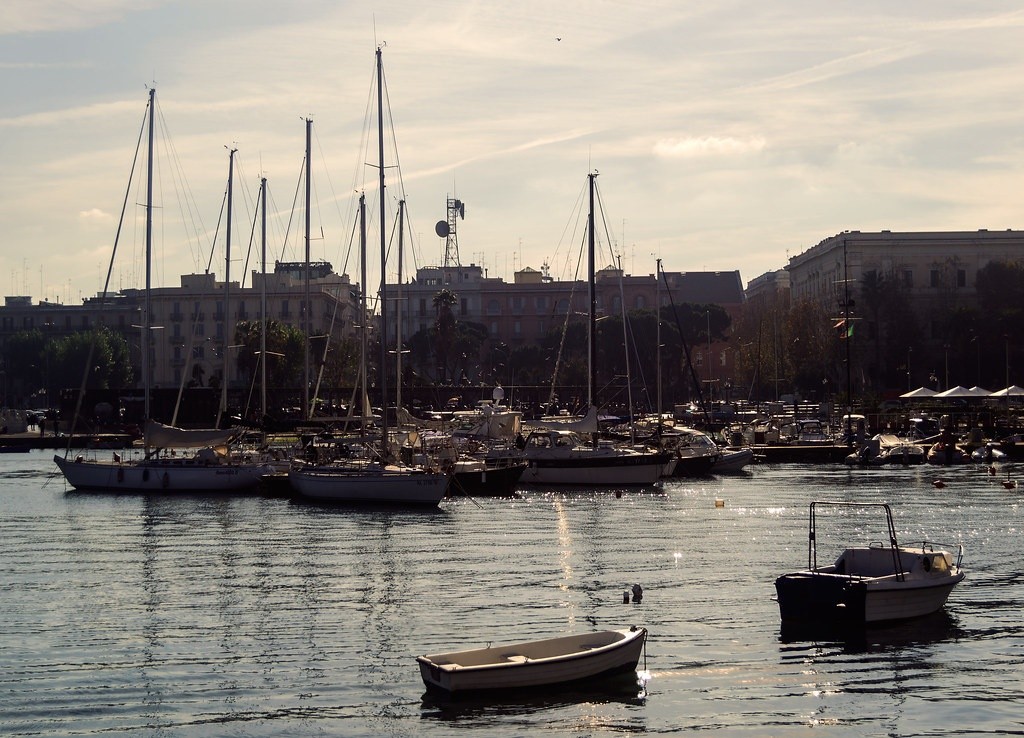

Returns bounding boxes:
[53,12,834,510]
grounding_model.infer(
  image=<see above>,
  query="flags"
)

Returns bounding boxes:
[840,320,855,339]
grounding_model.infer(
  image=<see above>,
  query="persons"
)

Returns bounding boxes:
[39,419,46,438]
[53,420,60,436]
[515,432,525,448]
[30,413,36,431]
[94,416,100,435]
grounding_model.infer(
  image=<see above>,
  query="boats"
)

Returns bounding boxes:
[972,445,1010,463]
[888,445,925,466]
[845,446,888,465]
[927,443,972,465]
[775,501,963,631]
[415,625,648,700]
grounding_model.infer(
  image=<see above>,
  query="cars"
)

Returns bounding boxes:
[35,408,49,414]
[25,410,34,417]
[33,411,46,420]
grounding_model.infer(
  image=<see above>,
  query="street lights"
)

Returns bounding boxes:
[971,337,980,388]
[1004,334,1010,429]
[907,347,912,393]
[944,344,950,390]
